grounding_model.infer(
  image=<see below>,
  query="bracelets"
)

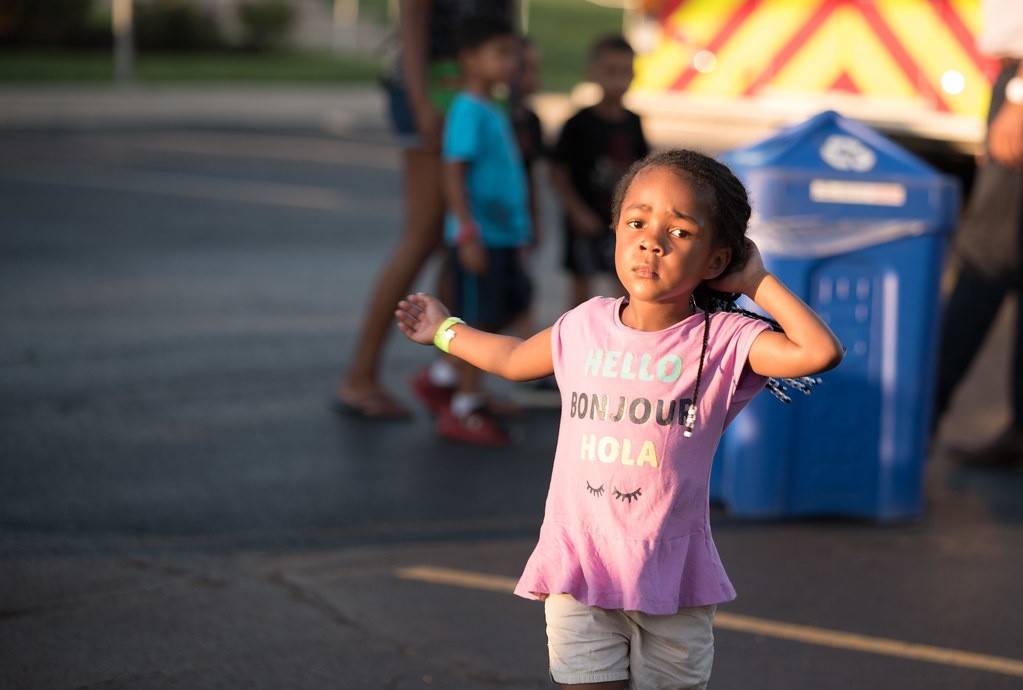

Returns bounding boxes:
[1004,77,1023,107]
[434,317,466,353]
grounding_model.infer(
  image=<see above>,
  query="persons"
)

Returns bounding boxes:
[335,0,654,454]
[927,0,1023,473]
[395,150,844,690]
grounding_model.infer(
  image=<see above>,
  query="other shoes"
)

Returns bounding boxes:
[440,407,513,448]
[952,422,1022,472]
[330,378,408,422]
[413,366,454,409]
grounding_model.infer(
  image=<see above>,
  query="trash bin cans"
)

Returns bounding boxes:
[709,109,959,521]
